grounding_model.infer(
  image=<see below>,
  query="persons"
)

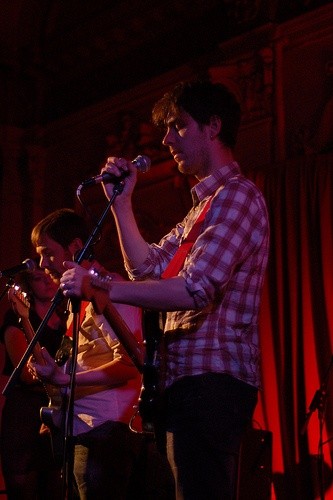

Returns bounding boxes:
[59,80,270,500]
[0,259,67,500]
[27,208,144,500]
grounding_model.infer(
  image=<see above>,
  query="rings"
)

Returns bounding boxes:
[61,281,67,289]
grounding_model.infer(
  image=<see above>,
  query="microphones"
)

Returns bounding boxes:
[82,155,151,186]
[0,259,36,278]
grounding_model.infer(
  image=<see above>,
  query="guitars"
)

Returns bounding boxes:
[5,273,76,465]
[81,265,187,468]
